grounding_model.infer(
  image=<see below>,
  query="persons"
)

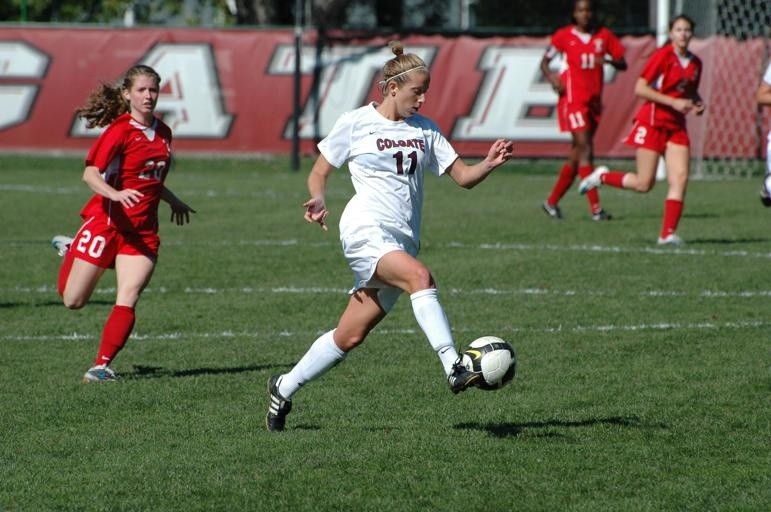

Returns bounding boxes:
[540,1,628,221]
[262,40,514,433]
[50,65,197,383]
[754,62,771,206]
[578,14,706,247]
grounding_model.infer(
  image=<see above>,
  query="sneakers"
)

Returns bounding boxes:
[51,234,76,256]
[542,200,563,218]
[760,171,771,208]
[578,163,611,195]
[264,374,293,434]
[82,365,120,383]
[592,209,613,222]
[448,359,481,396]
[657,234,685,248]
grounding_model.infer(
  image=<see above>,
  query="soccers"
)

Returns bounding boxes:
[462,336,515,390]
